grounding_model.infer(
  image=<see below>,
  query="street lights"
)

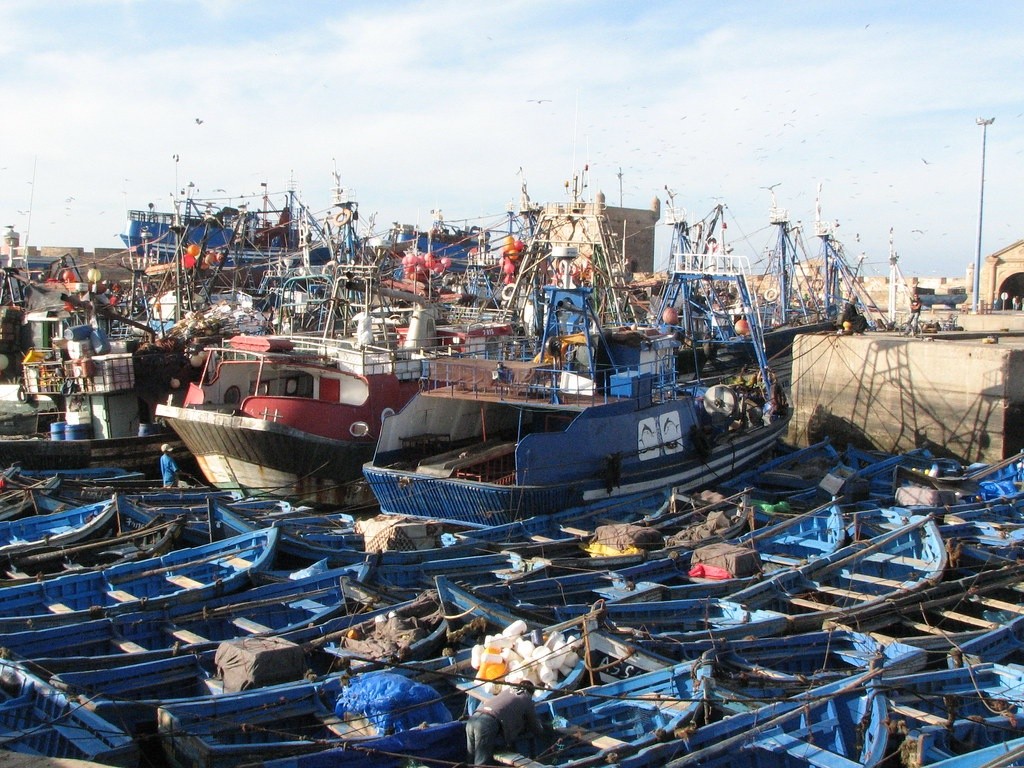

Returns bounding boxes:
[971,115,996,312]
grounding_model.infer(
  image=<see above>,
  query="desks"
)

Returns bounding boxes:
[400,433,452,453]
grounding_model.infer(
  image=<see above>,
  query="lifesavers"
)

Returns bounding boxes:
[61,381,74,397]
[334,209,350,226]
[763,288,777,301]
[501,283,519,300]
[16,386,31,404]
[322,260,339,276]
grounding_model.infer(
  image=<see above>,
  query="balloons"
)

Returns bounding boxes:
[87,268,101,282]
[402,253,451,280]
[499,236,524,286]
[181,244,225,270]
[62,271,75,280]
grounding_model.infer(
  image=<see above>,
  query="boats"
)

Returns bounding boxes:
[0,150,1024,768]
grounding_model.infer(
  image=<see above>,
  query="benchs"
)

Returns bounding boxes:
[523,532,552,541]
[164,575,205,590]
[314,710,367,742]
[104,589,138,604]
[443,676,495,703]
[549,717,630,751]
[42,600,74,614]
[2,562,32,580]
[752,732,863,768]
[109,637,147,655]
[781,550,1024,664]
[627,700,680,717]
[211,553,253,573]
[677,573,715,584]
[229,616,275,634]
[491,747,547,768]
[757,552,801,565]
[891,704,949,726]
[162,624,211,644]
[559,527,591,537]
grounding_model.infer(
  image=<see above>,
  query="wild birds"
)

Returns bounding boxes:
[520,21,935,237]
[0,115,204,241]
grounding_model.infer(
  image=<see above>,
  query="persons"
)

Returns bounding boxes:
[160,444,194,487]
[466,681,537,768]
[993,296,1024,310]
[843,295,868,333]
[905,293,922,335]
[495,362,514,384]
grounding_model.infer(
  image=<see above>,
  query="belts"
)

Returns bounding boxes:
[473,711,500,725]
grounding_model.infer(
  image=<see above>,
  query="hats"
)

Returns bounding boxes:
[161,444,173,452]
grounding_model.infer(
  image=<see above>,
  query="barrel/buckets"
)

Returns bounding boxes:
[91,330,111,355]
[137,423,160,437]
[50,422,68,440]
[65,424,91,440]
[64,324,92,341]
